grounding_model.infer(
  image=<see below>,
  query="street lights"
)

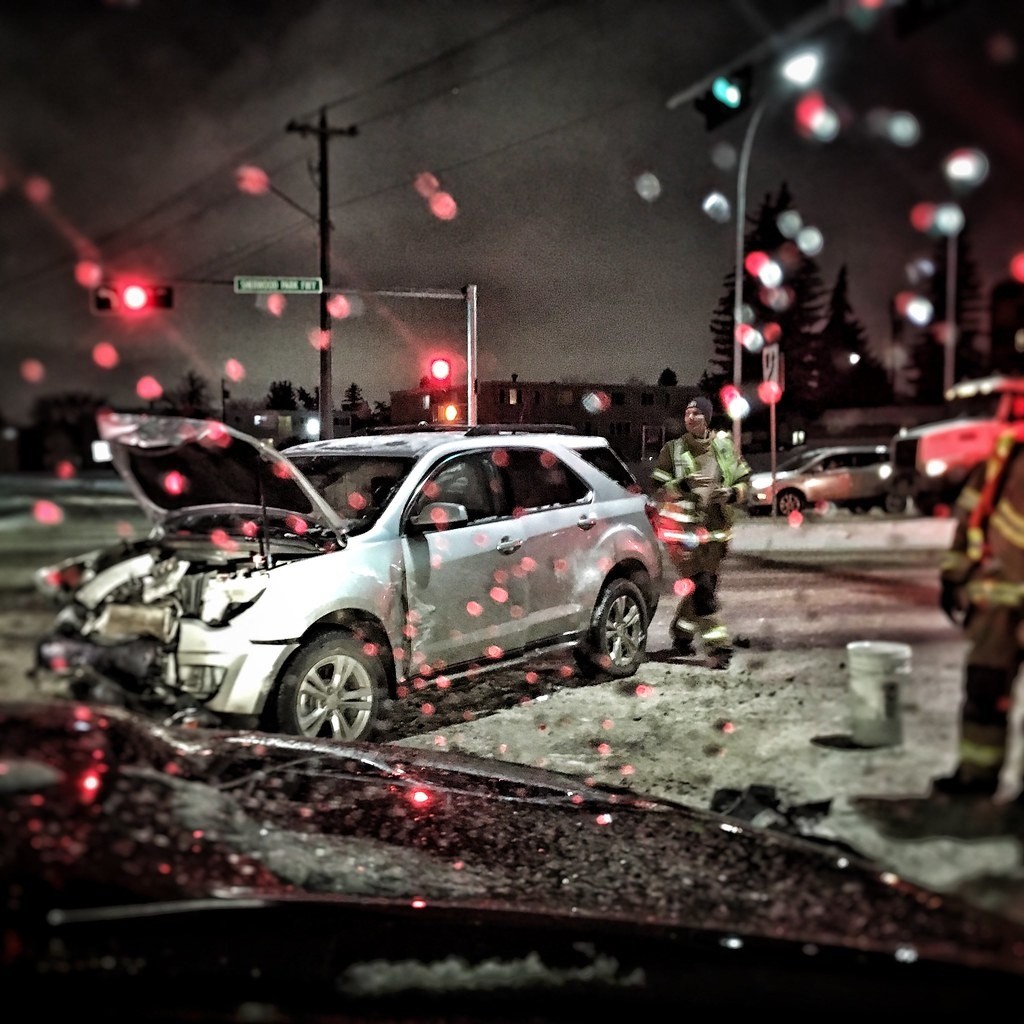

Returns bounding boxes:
[731,51,829,535]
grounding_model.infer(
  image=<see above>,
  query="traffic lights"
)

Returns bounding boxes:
[90,286,174,317]
[432,358,461,425]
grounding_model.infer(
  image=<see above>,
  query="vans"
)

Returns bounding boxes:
[891,417,1024,518]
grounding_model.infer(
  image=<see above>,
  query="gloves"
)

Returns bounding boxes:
[685,470,713,490]
[708,486,734,504]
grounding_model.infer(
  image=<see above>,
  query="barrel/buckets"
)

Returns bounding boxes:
[846,642,914,747]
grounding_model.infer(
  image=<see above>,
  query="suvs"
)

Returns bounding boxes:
[66,403,674,738]
[749,446,906,514]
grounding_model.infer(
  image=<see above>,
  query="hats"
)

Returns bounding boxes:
[687,396,712,427]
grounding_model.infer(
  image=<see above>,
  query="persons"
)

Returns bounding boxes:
[929,368,1023,833]
[641,397,755,668]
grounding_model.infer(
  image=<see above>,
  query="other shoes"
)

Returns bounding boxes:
[932,766,999,802]
[710,645,734,662]
[672,638,697,656]
